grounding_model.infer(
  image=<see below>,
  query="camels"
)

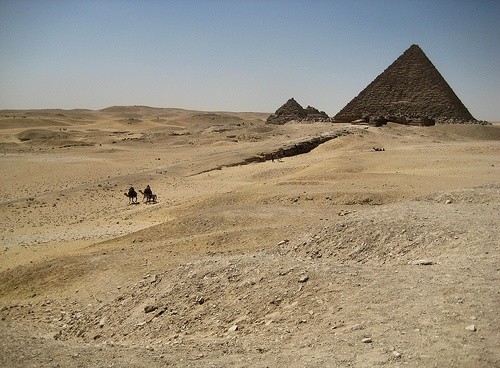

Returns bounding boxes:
[124,191,137,204]
[138,189,157,204]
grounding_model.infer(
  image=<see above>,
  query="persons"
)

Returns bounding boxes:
[128,187,134,198]
[144,185,152,199]
[380,148,385,151]
